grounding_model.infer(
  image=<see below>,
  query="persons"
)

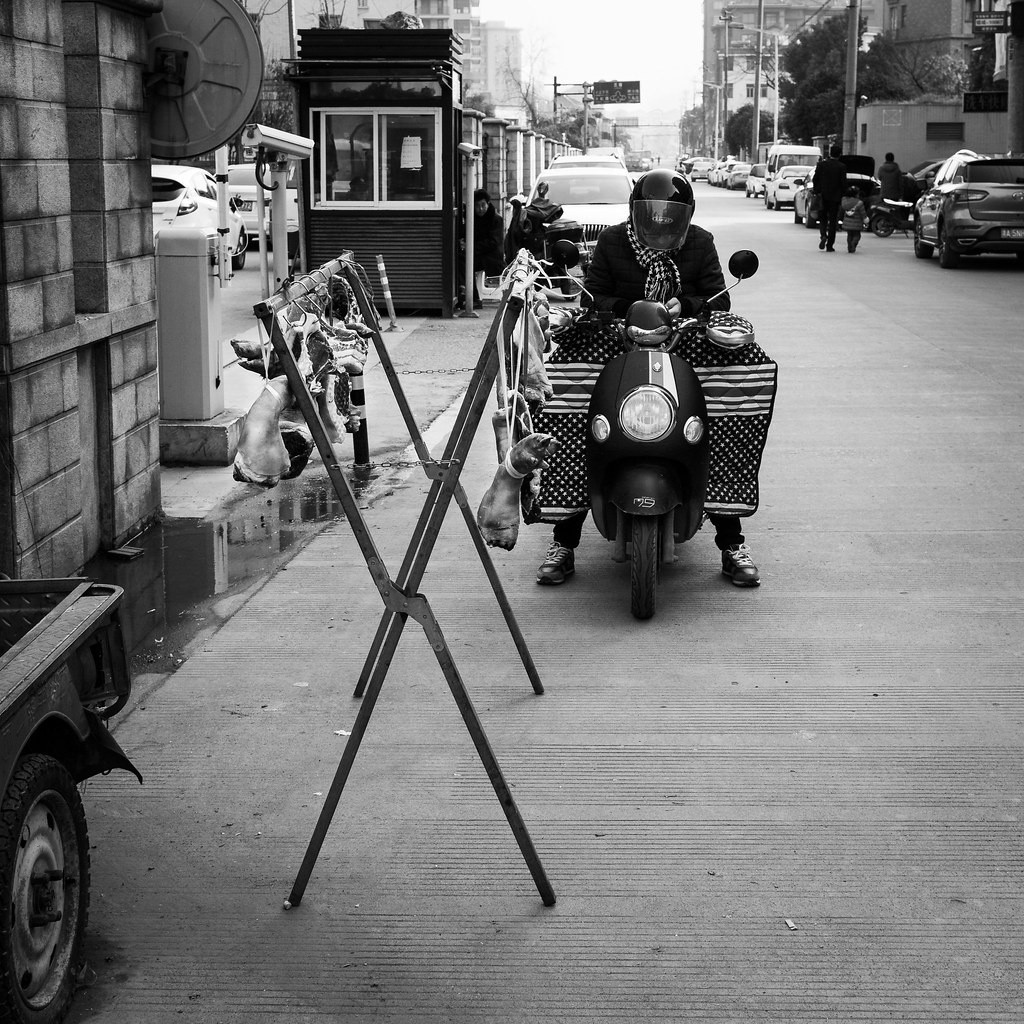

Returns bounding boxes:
[535,157,760,587]
[812,145,869,253]
[878,153,901,203]
[455,189,505,310]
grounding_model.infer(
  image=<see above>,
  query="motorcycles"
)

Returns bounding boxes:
[501,181,592,302]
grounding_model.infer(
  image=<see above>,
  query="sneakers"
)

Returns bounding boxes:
[536,541,575,585]
[722,543,761,586]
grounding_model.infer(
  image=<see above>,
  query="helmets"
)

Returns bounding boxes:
[630,169,695,251]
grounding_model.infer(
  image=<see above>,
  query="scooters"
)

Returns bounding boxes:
[550,248,759,620]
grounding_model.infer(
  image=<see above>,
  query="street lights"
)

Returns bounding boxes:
[728,21,778,143]
[702,82,722,160]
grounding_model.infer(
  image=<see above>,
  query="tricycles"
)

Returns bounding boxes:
[0,574,146,1024]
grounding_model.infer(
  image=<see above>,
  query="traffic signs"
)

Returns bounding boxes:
[590,80,642,104]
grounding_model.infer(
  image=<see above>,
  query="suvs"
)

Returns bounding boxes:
[509,152,635,265]
[870,148,1024,268]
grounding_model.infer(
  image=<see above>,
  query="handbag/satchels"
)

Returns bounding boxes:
[807,190,823,211]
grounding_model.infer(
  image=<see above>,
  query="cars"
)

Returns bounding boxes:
[707,160,752,190]
[765,144,823,211]
[682,156,717,182]
[151,163,299,275]
[794,154,881,228]
[746,164,767,197]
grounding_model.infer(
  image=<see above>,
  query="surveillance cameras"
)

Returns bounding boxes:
[458,143,482,159]
[242,123,315,159]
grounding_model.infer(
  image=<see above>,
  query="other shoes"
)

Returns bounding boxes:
[454,300,483,309]
[819,237,826,250]
[827,247,835,252]
